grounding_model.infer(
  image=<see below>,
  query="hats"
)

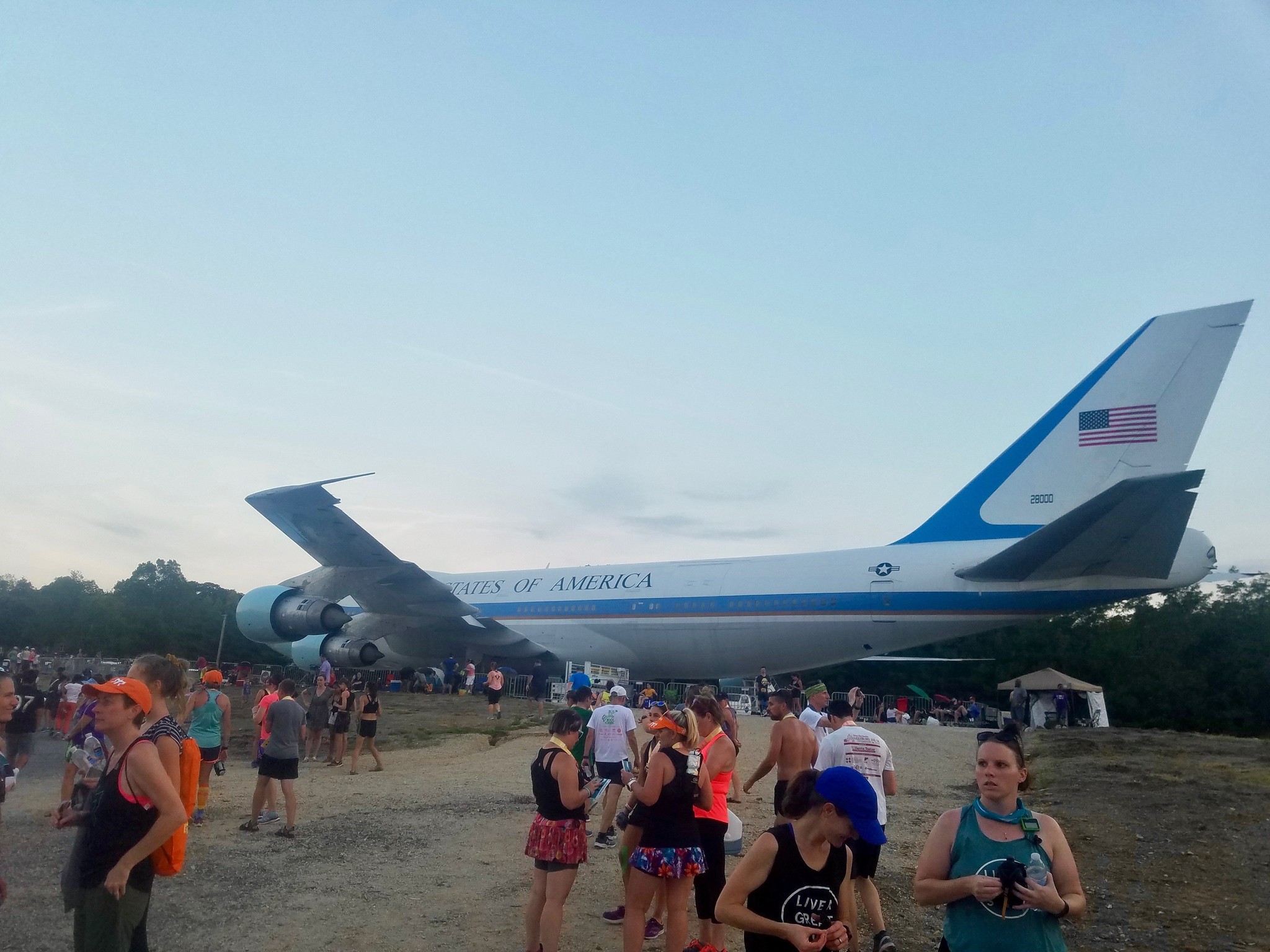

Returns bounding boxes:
[81,677,98,686]
[647,716,687,735]
[609,684,630,700]
[204,669,223,686]
[80,678,154,716]
[816,765,888,843]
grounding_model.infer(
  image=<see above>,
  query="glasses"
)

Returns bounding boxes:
[662,709,674,723]
[578,730,583,739]
[976,731,1025,761]
[651,702,668,710]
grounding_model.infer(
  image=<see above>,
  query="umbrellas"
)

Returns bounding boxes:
[419,667,434,675]
[907,685,951,703]
[399,667,416,678]
[497,666,518,678]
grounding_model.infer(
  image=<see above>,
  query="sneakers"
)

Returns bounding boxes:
[258,811,281,823]
[644,916,663,940]
[595,825,618,848]
[871,933,896,952]
[683,937,726,952]
[602,904,627,924]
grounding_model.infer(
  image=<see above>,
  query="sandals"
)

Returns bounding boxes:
[239,820,260,831]
[273,824,295,839]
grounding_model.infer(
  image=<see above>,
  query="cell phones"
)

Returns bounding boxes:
[583,775,603,788]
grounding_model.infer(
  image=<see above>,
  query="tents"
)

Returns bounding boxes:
[996,668,1109,728]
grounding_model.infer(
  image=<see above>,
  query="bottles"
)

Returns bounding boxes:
[70,746,98,775]
[686,747,702,777]
[1026,853,1047,913]
[83,732,107,771]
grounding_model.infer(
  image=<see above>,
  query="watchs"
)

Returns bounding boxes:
[843,925,853,942]
[627,780,637,792]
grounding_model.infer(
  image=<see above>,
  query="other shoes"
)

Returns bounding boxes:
[349,769,360,775]
[251,760,259,768]
[369,764,384,772]
[302,755,343,768]
[584,813,593,837]
[487,711,502,720]
[192,816,202,827]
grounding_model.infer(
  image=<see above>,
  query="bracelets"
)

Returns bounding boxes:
[583,755,588,759]
[67,734,71,740]
[252,711,257,716]
[854,703,861,710]
[583,787,591,796]
[625,804,631,813]
[626,803,633,811]
[629,778,635,782]
[221,746,228,750]
[1054,898,1069,918]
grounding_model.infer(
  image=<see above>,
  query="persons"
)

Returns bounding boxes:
[523,659,742,952]
[715,770,887,952]
[0,645,383,838]
[400,653,504,720]
[914,732,1085,952]
[0,672,18,906]
[742,665,896,952]
[50,655,191,952]
[1010,679,1071,729]
[886,696,979,728]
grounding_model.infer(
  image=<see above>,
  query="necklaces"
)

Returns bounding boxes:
[993,820,1011,840]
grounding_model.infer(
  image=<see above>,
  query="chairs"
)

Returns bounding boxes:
[661,689,681,710]
[635,691,658,708]
[1087,709,1100,728]
[959,708,980,729]
[619,683,634,708]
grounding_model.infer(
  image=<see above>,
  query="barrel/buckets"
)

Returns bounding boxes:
[390,680,402,692]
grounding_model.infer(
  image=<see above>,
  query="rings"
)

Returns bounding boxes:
[837,938,842,945]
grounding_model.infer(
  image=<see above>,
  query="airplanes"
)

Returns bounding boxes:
[234,298,1255,677]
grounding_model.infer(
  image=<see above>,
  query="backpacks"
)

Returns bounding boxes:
[128,720,201,876]
